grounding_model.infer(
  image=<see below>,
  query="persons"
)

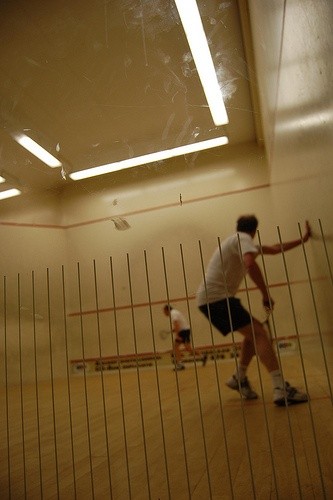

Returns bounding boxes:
[198,215,312,406]
[160,305,206,370]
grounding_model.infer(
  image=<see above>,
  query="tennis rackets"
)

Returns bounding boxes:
[262,309,275,342]
[159,328,176,340]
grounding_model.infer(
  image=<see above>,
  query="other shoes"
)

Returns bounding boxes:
[173,366,185,371]
[202,355,207,367]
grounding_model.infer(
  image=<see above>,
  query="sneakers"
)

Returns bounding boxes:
[272,380,307,406]
[226,373,258,400]
